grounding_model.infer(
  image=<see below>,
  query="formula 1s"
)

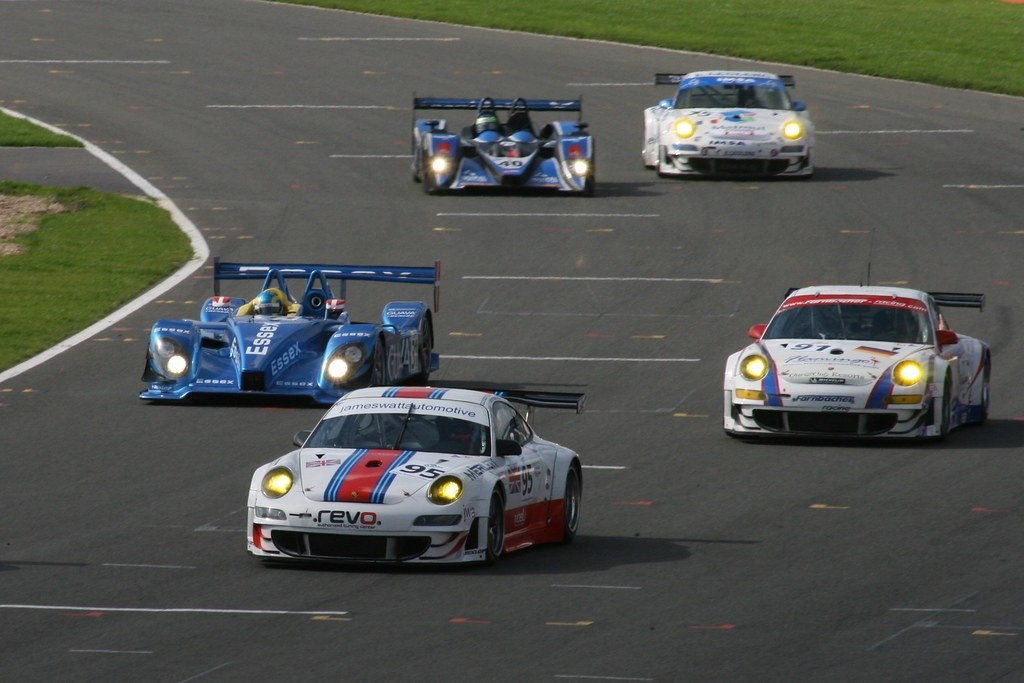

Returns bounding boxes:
[407,97,598,194]
[139,257,440,409]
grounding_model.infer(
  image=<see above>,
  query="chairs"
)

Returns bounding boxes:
[873,308,918,342]
[295,270,333,319]
[380,415,440,451]
[237,268,303,317]
[460,96,535,139]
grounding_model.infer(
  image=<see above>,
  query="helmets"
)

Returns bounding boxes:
[434,415,474,447]
[474,113,501,136]
[251,290,283,316]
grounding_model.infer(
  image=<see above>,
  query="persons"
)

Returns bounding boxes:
[255,291,284,316]
[475,113,499,134]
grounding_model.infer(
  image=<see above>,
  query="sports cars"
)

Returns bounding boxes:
[642,68,818,179]
[247,378,588,572]
[723,280,995,442]
